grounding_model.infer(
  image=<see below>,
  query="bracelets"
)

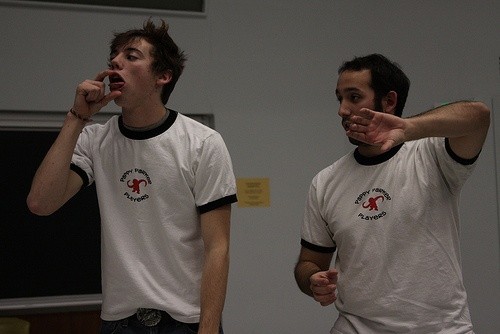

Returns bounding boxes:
[70,108,92,122]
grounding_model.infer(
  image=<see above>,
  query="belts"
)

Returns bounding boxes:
[118,308,176,327]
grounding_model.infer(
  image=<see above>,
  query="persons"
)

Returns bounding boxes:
[294,53,491,332]
[26,16,239,334]
